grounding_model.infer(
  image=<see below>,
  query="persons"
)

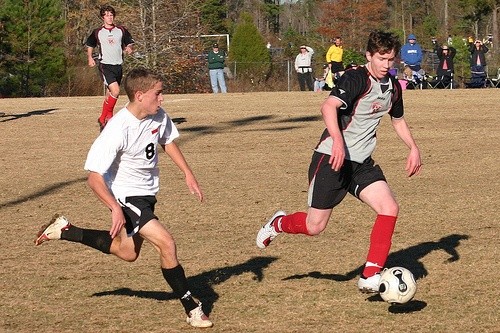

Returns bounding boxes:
[86,6,136,133]
[401,34,423,72]
[34,66,214,328]
[469,40,489,67]
[295,44,314,91]
[437,45,456,79]
[314,36,345,92]
[208,43,228,93]
[256,28,421,293]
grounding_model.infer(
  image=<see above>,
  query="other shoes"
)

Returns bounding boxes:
[98,118,108,133]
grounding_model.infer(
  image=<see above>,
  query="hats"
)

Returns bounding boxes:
[213,43,218,48]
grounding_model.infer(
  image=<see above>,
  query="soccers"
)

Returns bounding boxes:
[378,267,417,307]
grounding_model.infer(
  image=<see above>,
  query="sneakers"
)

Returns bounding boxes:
[256,210,287,249]
[358,268,388,293]
[33,212,71,246]
[186,301,212,327]
